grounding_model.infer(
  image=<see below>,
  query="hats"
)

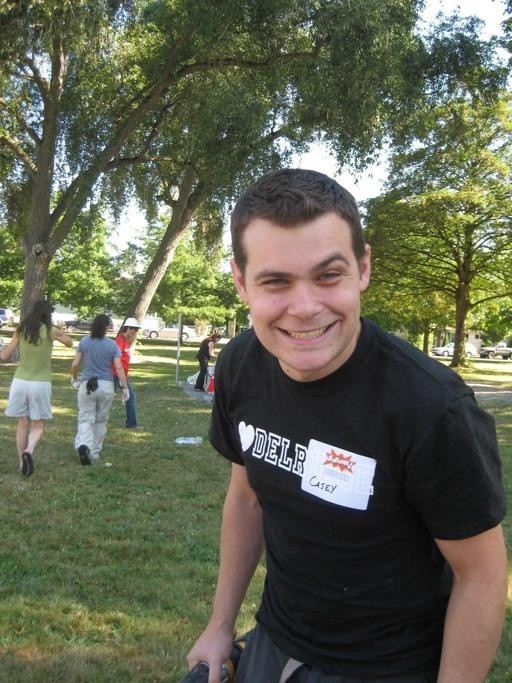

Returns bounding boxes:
[122,316,142,329]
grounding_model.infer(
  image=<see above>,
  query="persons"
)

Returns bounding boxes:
[68,312,132,466]
[0,298,75,478]
[181,166,512,682]
[192,332,223,392]
[110,314,146,432]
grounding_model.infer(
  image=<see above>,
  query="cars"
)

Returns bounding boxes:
[479,342,512,359]
[0,308,9,327]
[142,322,195,341]
[64,314,113,332]
[432,341,477,358]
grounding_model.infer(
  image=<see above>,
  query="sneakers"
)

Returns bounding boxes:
[78,444,91,466]
[22,451,34,477]
[193,386,205,392]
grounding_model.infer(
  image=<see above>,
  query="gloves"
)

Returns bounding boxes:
[71,378,81,389]
[122,388,130,406]
